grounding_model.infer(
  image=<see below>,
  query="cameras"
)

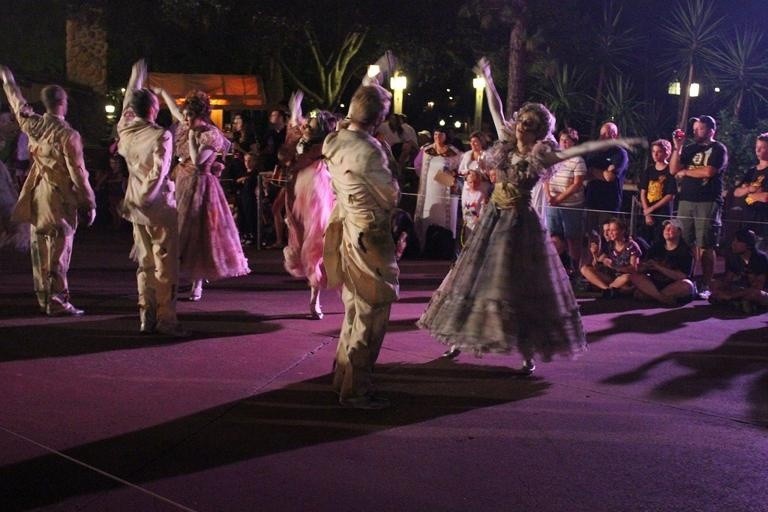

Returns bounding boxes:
[676,130,685,137]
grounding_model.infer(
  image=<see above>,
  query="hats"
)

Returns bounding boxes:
[689,114,716,125]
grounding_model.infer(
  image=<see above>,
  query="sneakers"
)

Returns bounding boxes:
[265,241,283,250]
[732,300,758,314]
[634,290,644,300]
[665,295,676,306]
[602,287,618,300]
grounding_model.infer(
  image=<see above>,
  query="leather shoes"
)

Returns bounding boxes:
[48,305,84,316]
[310,302,326,319]
[523,358,536,375]
[139,322,154,332]
[443,347,461,358]
[341,394,390,411]
[189,287,202,300]
[159,325,193,338]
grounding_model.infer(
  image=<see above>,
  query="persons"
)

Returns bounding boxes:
[153,87,251,302]
[225,90,408,318]
[375,110,768,316]
[415,58,644,377]
[117,57,193,339]
[0,65,97,316]
[323,55,400,410]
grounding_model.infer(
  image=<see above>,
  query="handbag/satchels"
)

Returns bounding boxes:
[425,224,454,260]
